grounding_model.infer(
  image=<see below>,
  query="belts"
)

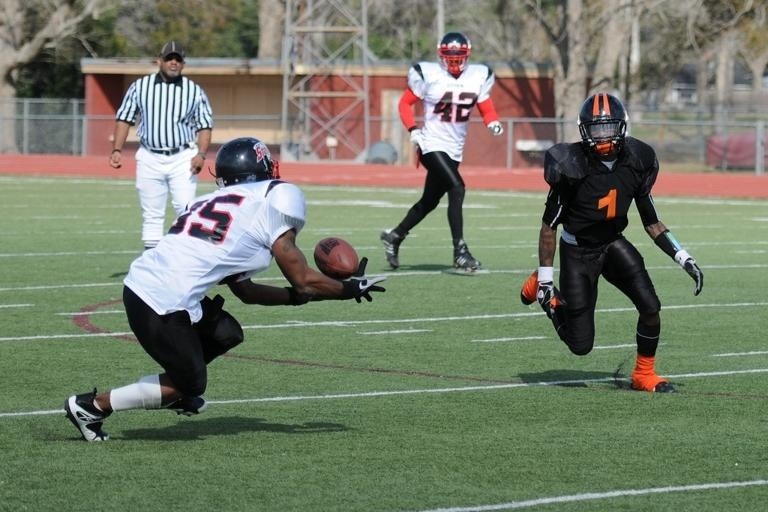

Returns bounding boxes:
[150,144,189,155]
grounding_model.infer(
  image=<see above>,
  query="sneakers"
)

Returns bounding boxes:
[454,244,481,267]
[64,387,110,442]
[380,228,408,269]
[163,396,207,417]
[631,374,676,393]
[521,270,538,305]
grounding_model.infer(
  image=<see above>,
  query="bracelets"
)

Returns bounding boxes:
[654,229,683,258]
[538,267,554,281]
[673,249,693,266]
[196,151,207,160]
[282,286,309,306]
[111,148,122,154]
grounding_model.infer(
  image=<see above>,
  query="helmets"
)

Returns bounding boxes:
[214,138,279,186]
[437,32,472,76]
[577,93,627,159]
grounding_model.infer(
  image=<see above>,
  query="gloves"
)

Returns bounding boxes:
[684,258,704,296]
[537,281,568,320]
[409,129,426,145]
[487,120,504,137]
[341,258,387,303]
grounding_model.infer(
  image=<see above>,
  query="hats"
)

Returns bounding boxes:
[159,40,185,61]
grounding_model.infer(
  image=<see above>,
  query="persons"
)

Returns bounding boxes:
[519,92,703,394]
[379,32,504,271]
[110,39,213,248]
[65,138,387,440]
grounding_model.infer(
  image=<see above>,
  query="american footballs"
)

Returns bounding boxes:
[314,238,358,280]
